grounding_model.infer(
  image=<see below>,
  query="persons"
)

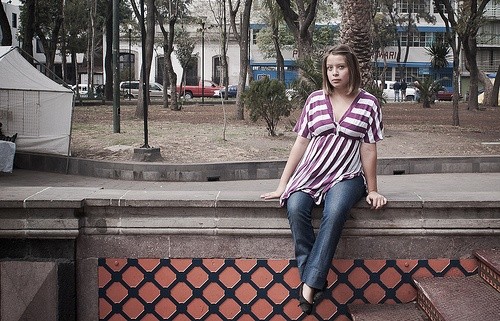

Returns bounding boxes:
[259,44,388,316]
[400,78,407,102]
[393,80,401,102]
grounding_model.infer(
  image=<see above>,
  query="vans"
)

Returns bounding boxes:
[119,80,172,98]
[374,80,420,102]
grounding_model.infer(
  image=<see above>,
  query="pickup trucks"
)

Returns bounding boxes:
[169,81,226,99]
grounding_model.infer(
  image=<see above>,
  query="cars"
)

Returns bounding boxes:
[220,85,251,97]
[285,89,298,100]
[428,86,454,101]
[70,84,97,97]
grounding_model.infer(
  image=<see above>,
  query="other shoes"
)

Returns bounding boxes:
[298,283,316,315]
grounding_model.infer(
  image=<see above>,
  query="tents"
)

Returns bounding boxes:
[0,45,76,174]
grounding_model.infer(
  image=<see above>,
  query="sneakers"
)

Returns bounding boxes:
[313,280,328,305]
